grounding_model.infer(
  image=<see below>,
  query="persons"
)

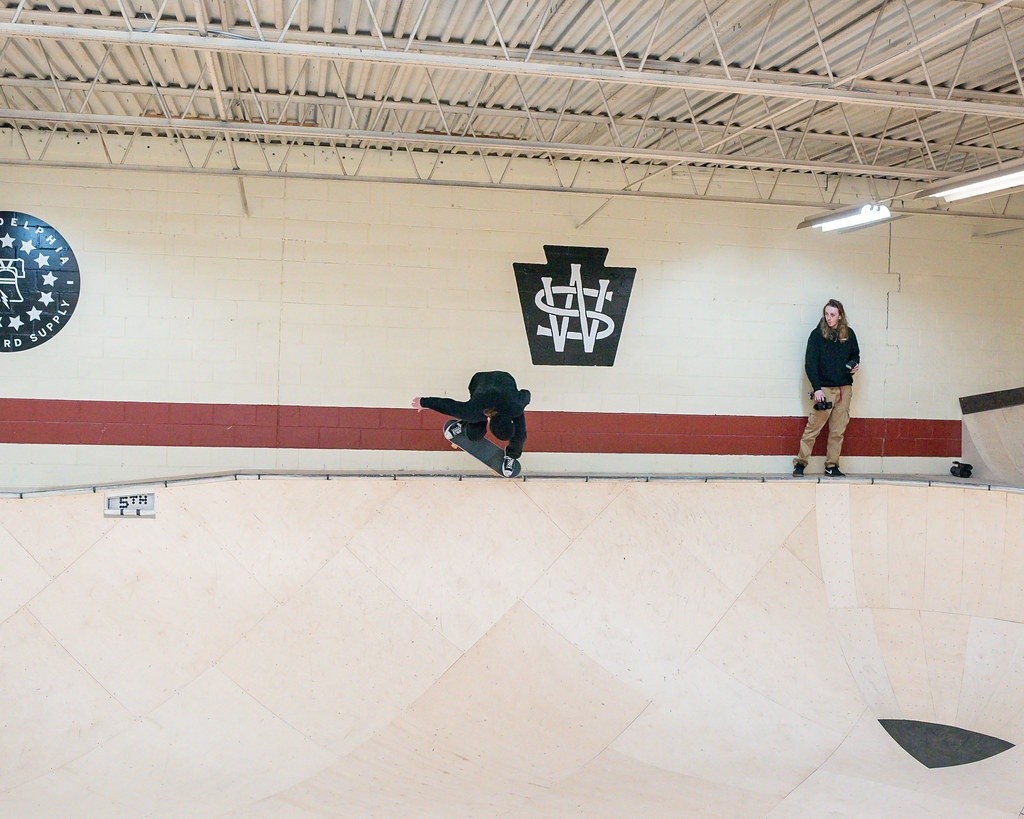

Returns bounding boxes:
[412,371,530,477]
[792,299,860,479]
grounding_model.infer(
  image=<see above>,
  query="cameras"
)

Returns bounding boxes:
[813,396,833,411]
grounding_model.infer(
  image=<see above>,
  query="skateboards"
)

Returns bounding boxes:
[442,418,521,478]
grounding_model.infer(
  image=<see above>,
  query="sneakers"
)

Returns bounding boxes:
[501,446,514,477]
[793,461,805,477]
[444,419,463,440]
[825,465,845,477]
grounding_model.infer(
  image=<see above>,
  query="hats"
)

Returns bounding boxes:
[492,415,515,440]
